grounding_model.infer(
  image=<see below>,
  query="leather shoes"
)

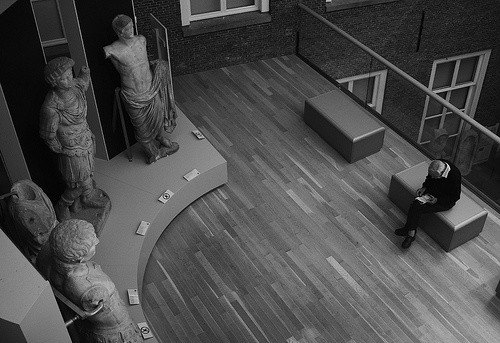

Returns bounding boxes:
[395,228,408,236]
[401,235,415,249]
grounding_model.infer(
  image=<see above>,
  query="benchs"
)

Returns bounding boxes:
[388,161,488,252]
[304,89,386,164]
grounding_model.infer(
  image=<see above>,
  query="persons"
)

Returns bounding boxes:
[7,57,139,343]
[103,15,180,165]
[395,158,461,249]
